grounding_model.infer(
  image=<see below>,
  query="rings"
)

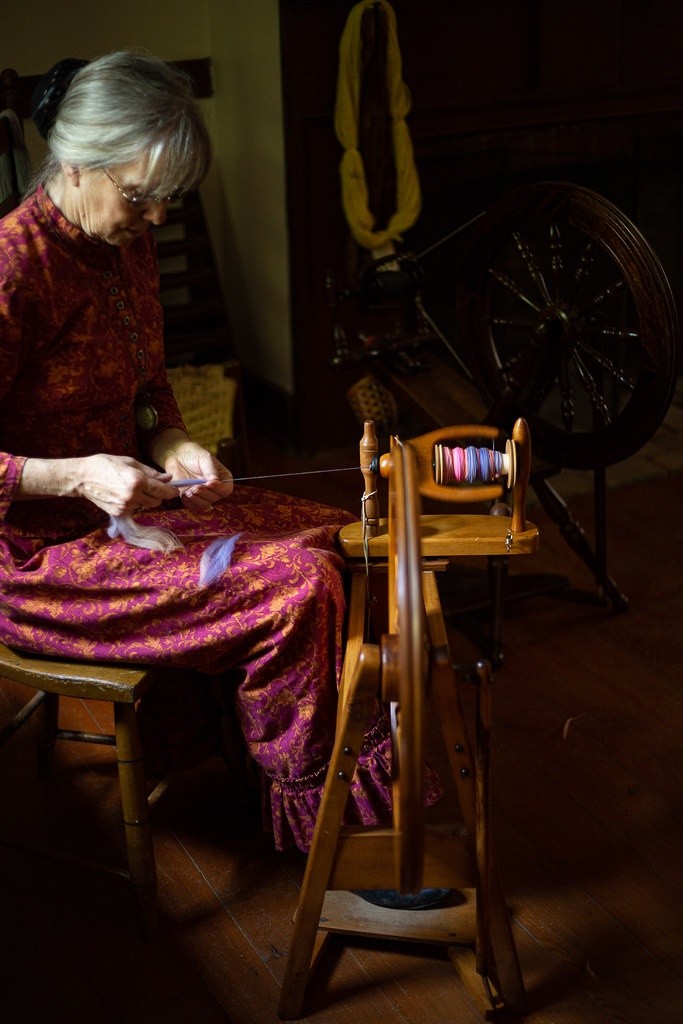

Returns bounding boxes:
[135,505,144,512]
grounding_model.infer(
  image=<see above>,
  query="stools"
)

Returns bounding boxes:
[1,646,256,941]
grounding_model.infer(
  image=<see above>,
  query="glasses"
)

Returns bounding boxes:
[102,167,185,208]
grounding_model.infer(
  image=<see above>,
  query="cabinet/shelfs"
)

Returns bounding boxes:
[280,0,683,457]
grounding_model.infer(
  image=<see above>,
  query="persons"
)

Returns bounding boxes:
[0,49,452,909]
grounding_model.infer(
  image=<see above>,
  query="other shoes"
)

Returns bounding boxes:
[350,881,453,909]
